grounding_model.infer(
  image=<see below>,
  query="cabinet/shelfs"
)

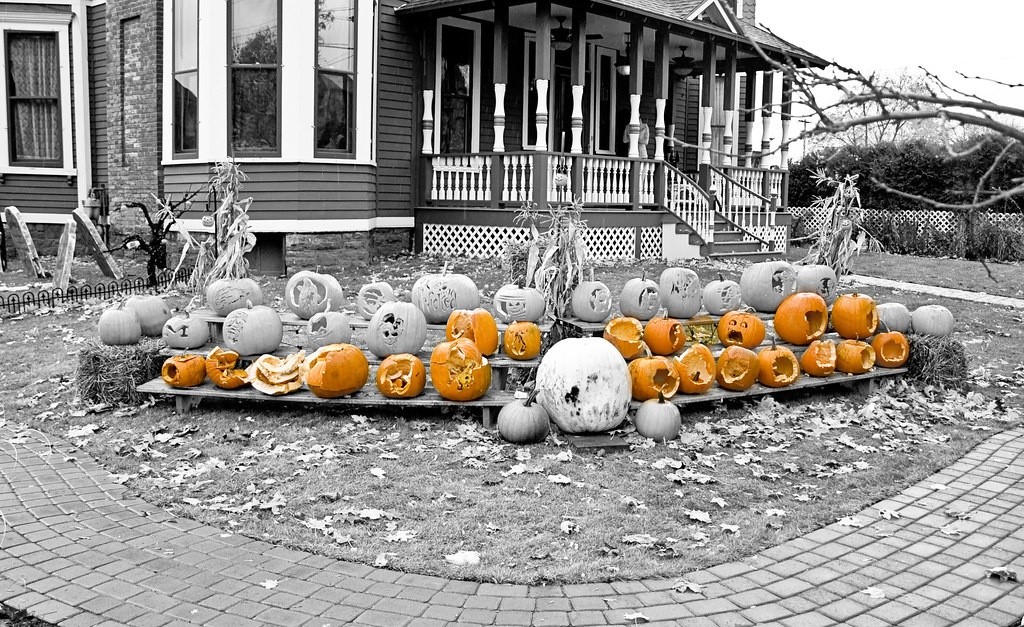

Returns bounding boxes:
[134,303,911,427]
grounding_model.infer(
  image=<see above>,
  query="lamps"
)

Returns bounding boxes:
[614,32,643,76]
[672,63,695,80]
[549,41,573,51]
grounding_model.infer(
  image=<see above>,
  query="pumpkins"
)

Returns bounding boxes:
[497,390,550,444]
[875,303,911,332]
[98,304,141,344]
[125,295,172,336]
[912,305,954,336]
[537,336,632,432]
[635,391,679,440]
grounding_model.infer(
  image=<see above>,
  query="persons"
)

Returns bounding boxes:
[623,113,649,159]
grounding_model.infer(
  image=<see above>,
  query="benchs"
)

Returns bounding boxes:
[647,46,720,65]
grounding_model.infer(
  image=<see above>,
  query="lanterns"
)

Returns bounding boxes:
[357,281,394,319]
[573,281,612,321]
[619,278,661,320]
[366,301,426,357]
[661,268,701,318]
[412,274,480,324]
[741,261,797,311]
[162,315,209,348]
[717,311,764,350]
[118,294,170,336]
[305,311,352,351]
[493,285,545,323]
[98,307,141,346]
[702,280,741,315]
[285,271,344,320]
[221,305,283,355]
[206,279,261,316]
[797,265,837,306]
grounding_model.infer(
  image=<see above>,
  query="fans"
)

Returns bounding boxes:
[524,16,602,41]
